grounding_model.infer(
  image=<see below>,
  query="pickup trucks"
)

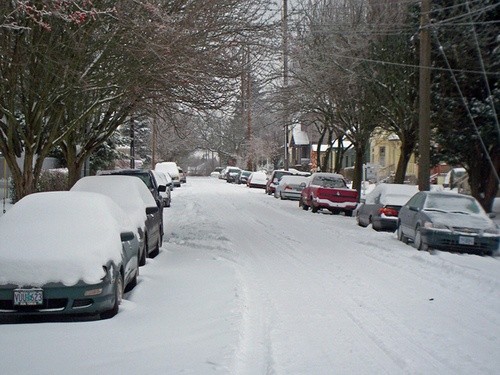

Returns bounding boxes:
[299,172,359,217]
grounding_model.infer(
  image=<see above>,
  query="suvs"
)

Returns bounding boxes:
[266,170,294,195]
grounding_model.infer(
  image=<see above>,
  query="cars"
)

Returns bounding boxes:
[0,191,140,320]
[70,175,164,266]
[96,168,166,213]
[151,161,188,207]
[275,174,307,201]
[210,166,273,189]
[380,167,469,190]
[356,182,419,232]
[397,189,500,256]
